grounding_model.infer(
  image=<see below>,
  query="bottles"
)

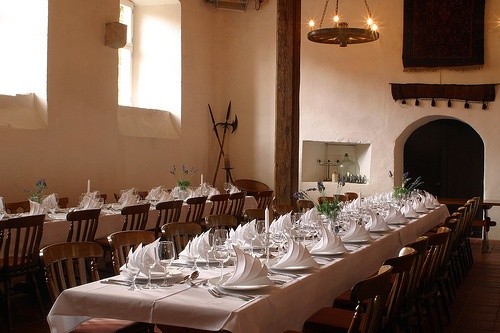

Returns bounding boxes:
[338,153,355,181]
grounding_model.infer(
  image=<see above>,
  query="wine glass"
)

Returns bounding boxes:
[41,186,427,295]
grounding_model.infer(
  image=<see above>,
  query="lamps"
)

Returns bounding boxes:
[308,0,380,48]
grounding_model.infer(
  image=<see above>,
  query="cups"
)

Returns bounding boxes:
[331,174,338,182]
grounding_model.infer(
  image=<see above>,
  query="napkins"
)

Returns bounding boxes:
[80,196,100,211]
[45,192,57,210]
[28,200,43,216]
[106,188,438,296]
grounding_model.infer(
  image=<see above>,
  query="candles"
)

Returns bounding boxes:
[201,173,203,184]
[359,193,361,207]
[87,179,90,193]
[264,205,270,231]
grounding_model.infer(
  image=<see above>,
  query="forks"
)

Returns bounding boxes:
[208,287,255,302]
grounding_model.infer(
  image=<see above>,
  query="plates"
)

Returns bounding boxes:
[270,263,319,270]
[428,203,441,210]
[220,280,275,290]
[309,248,349,255]
[406,214,418,219]
[417,209,428,213]
[341,238,374,244]
[369,227,395,233]
[386,221,408,225]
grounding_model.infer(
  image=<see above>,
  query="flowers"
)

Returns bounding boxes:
[25,178,49,206]
[170,165,198,186]
[387,170,424,204]
[295,184,348,222]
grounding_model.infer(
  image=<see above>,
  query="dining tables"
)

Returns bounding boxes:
[371,202,449,244]
[1,197,258,268]
[47,228,399,332]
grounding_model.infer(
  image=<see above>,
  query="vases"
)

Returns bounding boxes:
[178,186,190,201]
[397,199,405,211]
[330,218,341,236]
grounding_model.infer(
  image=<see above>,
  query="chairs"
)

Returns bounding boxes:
[1,186,358,299]
[302,197,482,333]
